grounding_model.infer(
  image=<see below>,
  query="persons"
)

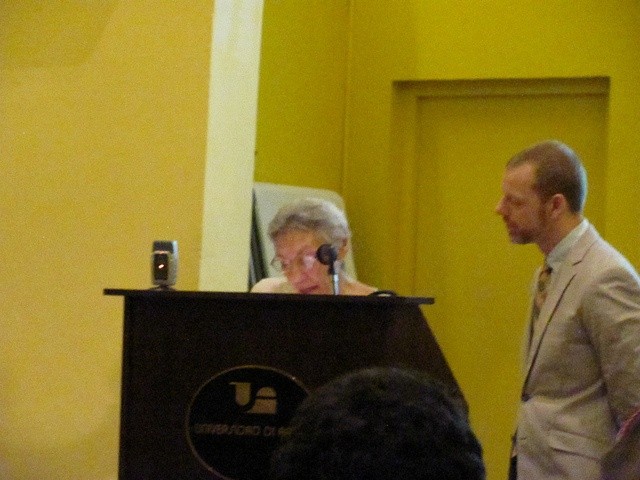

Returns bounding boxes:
[247,197,391,297]
[495,140,639,480]
[271,364,487,480]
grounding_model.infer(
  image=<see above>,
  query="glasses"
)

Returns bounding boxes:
[271,251,319,277]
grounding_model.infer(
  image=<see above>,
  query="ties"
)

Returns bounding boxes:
[528,257,552,359]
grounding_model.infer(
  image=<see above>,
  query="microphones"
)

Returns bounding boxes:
[317,244,339,263]
